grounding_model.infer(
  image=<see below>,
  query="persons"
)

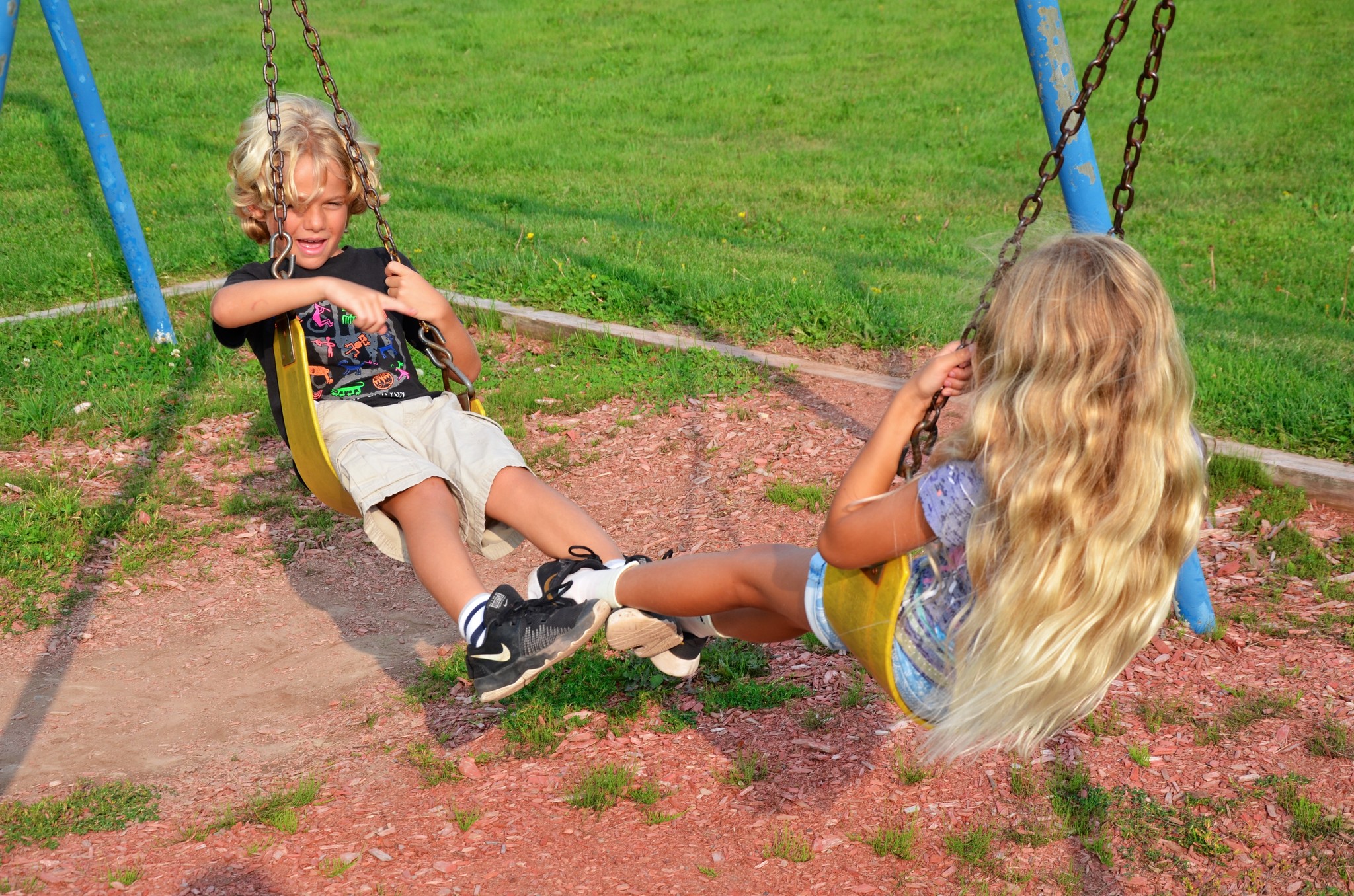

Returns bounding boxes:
[210,91,684,704]
[526,232,1212,767]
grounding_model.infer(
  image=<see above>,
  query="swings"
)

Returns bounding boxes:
[258,0,488,514]
[825,0,1179,733]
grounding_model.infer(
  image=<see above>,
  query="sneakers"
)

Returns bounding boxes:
[623,550,709,677]
[605,607,683,658]
[465,584,609,704]
[527,557,606,608]
